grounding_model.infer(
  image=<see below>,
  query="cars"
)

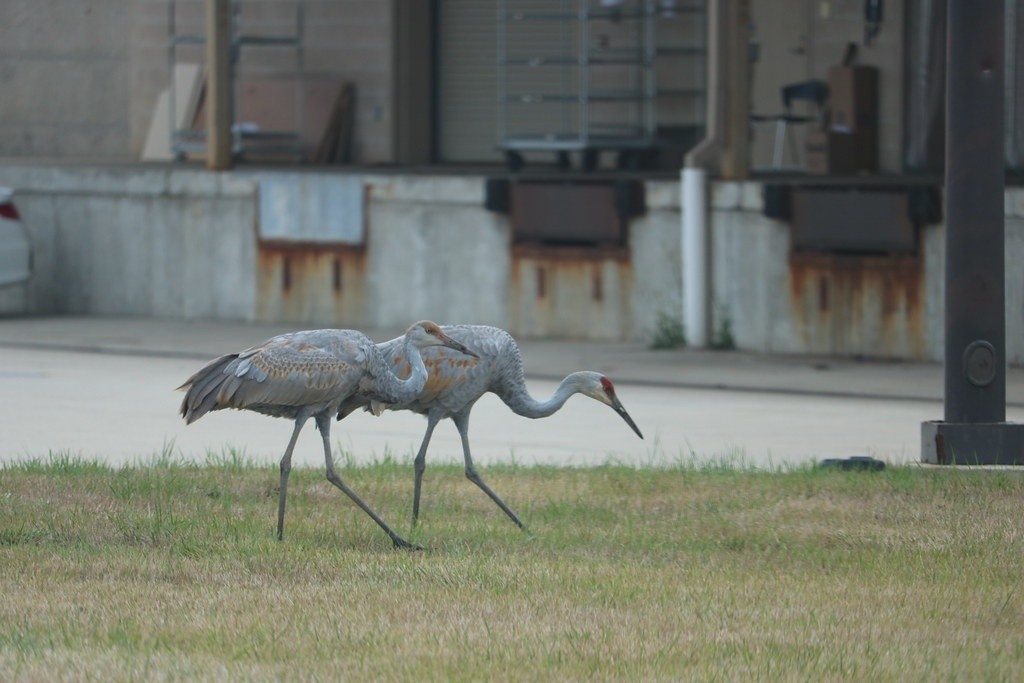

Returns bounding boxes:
[0,187,33,286]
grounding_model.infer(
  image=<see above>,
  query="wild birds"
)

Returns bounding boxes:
[170,320,645,554]
